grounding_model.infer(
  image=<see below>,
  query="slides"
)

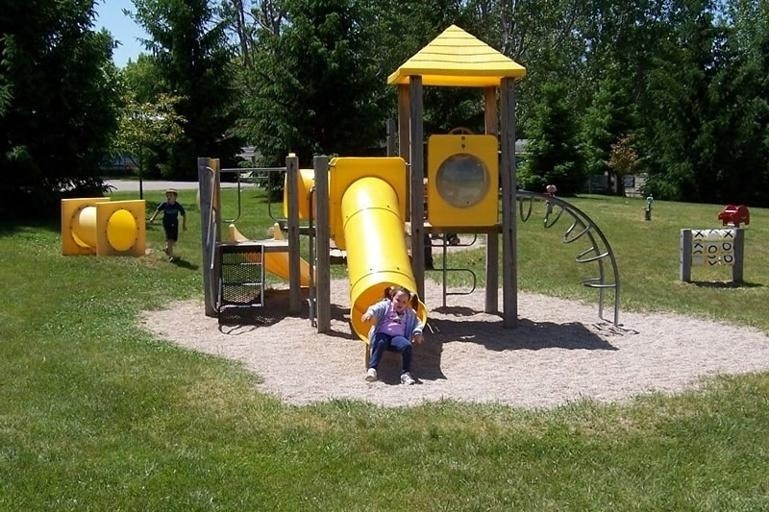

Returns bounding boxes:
[230,221,316,289]
[341,176,428,351]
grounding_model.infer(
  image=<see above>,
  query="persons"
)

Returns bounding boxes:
[421,175,459,271]
[359,283,427,386]
[148,186,187,264]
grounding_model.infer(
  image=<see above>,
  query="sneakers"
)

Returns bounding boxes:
[365,368,378,382]
[163,247,174,263]
[400,372,415,385]
